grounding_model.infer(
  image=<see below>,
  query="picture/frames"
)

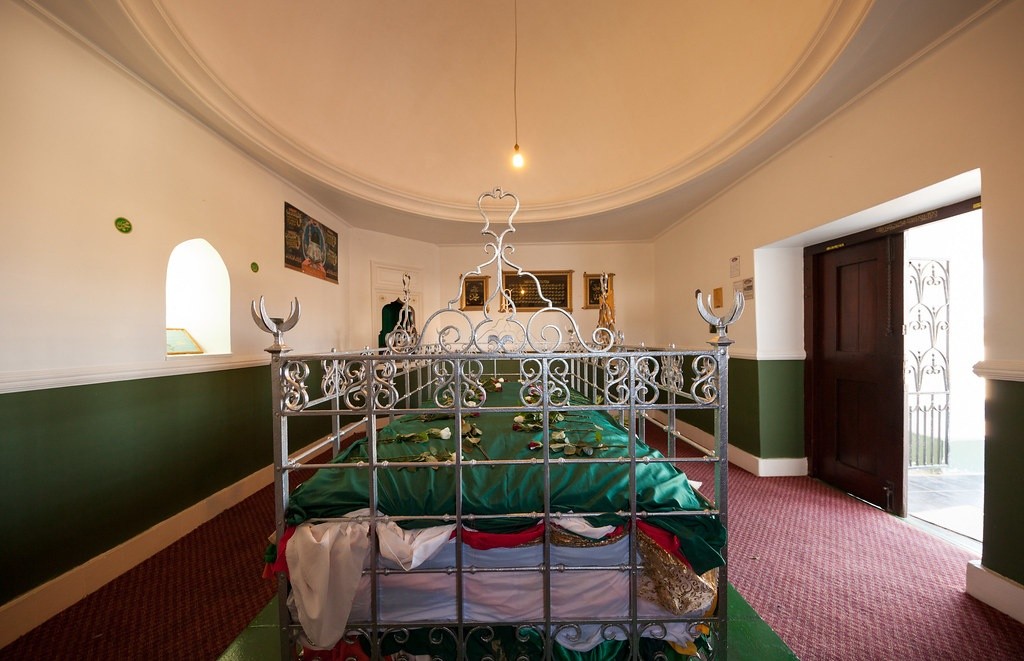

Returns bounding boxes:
[498,270,575,314]
[166,328,204,354]
[581,272,615,310]
[459,274,491,313]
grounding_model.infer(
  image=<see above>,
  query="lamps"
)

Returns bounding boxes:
[512,0,523,167]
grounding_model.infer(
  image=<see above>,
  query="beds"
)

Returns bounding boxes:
[251,185,744,661]
